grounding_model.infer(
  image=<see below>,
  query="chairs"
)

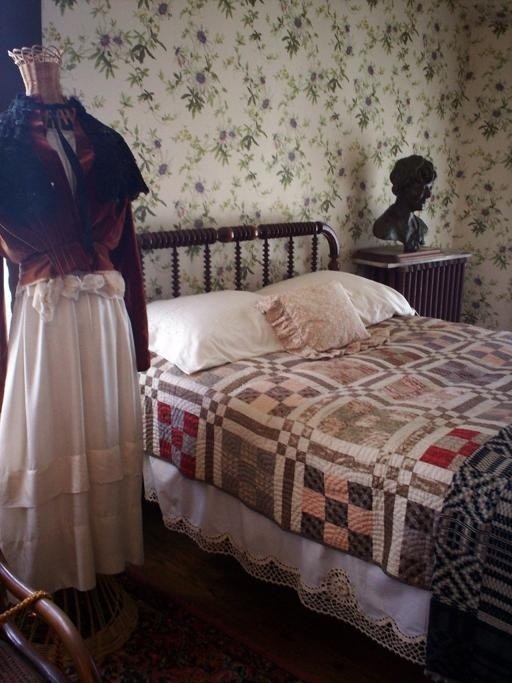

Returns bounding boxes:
[0,551,104,683]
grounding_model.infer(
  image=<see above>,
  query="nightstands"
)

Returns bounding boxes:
[354,249,473,322]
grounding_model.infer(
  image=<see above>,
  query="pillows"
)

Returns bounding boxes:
[146,290,284,377]
[255,282,389,358]
[259,270,420,327]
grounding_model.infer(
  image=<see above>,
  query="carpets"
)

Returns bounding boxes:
[13,573,305,683]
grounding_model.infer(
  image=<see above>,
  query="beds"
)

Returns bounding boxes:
[138,223,512,683]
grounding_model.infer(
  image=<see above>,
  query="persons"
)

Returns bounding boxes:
[0,44,150,609]
[373,155,436,252]
